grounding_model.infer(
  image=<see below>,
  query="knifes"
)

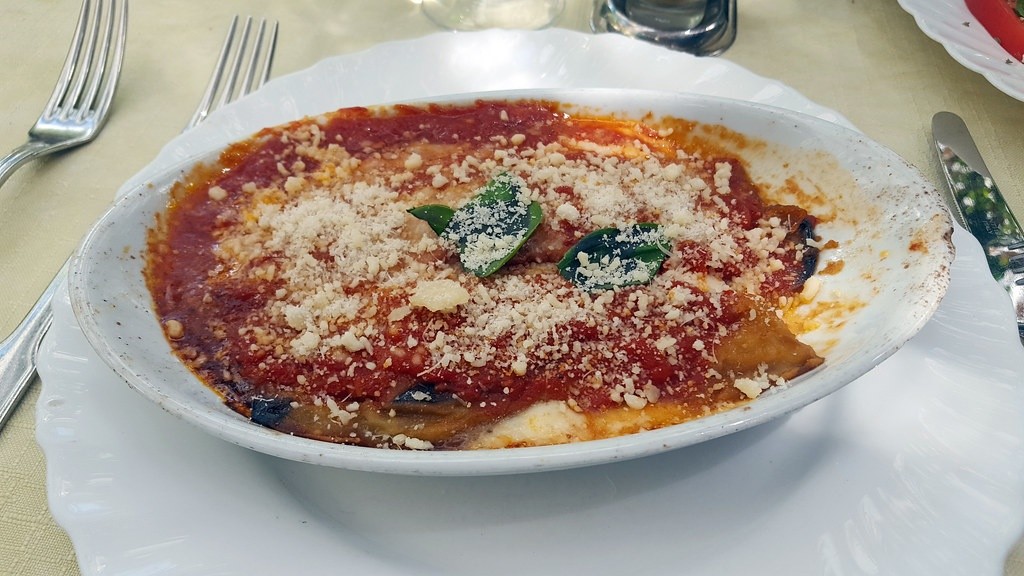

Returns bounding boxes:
[930,108,1023,342]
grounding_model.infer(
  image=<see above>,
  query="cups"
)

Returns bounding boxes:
[586,1,740,60]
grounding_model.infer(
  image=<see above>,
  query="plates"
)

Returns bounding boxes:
[62,89,955,475]
[892,0,1024,107]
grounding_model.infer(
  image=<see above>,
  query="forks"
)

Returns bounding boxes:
[0,1,135,198]
[0,15,287,445]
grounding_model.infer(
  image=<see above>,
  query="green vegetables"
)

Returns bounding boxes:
[404,172,673,288]
